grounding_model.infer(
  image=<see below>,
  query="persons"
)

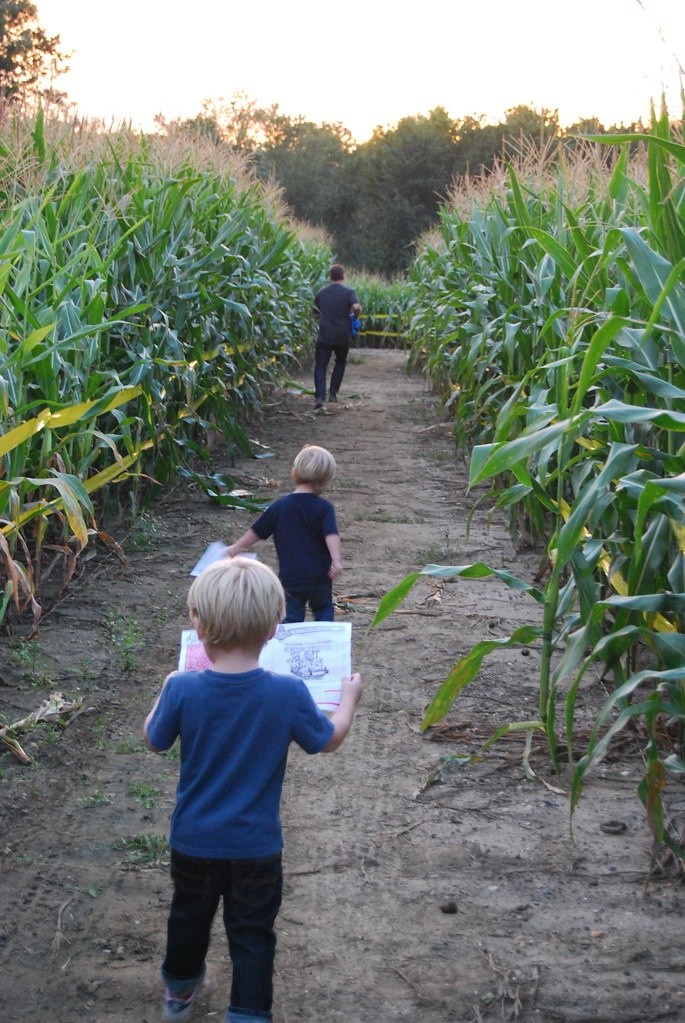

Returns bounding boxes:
[310,264,362,409]
[140,555,364,1022]
[222,445,343,622]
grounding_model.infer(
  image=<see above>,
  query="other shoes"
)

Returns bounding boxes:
[161,962,207,1021]
[315,397,323,409]
[329,395,338,402]
